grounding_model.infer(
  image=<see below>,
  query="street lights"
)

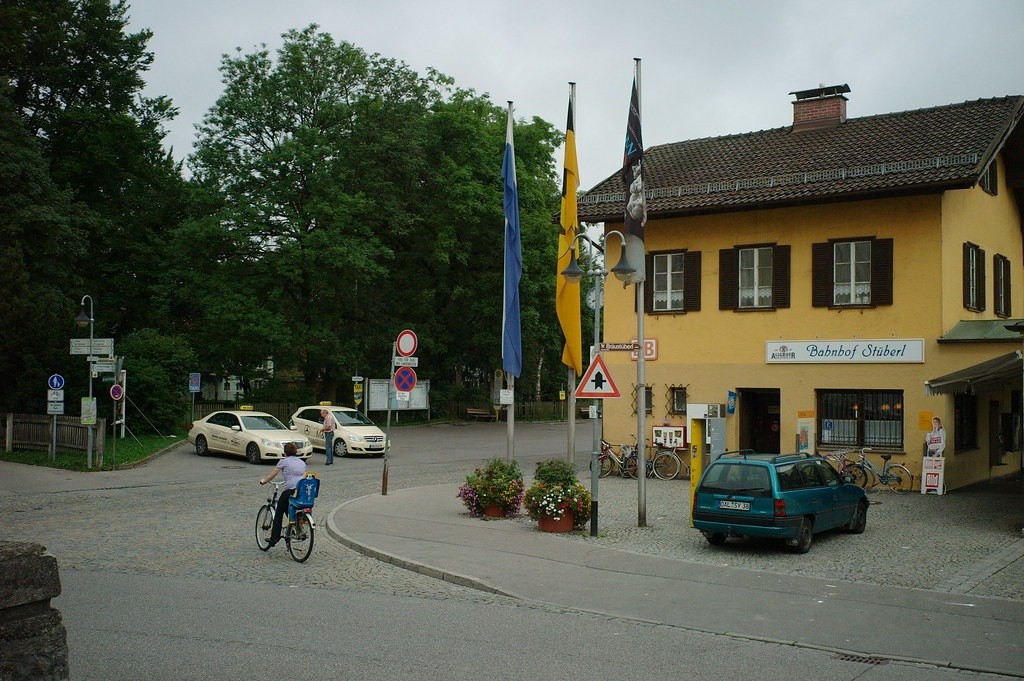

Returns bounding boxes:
[74,295,96,470]
[561,230,638,529]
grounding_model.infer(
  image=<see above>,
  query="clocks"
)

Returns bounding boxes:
[586,286,605,309]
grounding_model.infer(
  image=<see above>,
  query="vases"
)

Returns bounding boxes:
[538,511,573,532]
[486,505,507,517]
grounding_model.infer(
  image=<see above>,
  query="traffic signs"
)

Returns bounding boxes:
[352,377,364,381]
[90,358,117,373]
[69,339,113,355]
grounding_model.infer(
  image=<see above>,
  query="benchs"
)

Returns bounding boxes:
[466,408,496,422]
[580,407,600,418]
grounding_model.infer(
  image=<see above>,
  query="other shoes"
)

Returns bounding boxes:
[326,463,330,465]
[265,538,278,542]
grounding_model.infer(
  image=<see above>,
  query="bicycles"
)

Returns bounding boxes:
[255,480,317,562]
[799,448,914,495]
[590,432,691,480]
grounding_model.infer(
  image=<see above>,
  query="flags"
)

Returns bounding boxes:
[498,101,523,378]
[555,82,584,377]
[613,61,647,288]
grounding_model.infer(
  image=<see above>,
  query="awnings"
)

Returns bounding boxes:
[924,349,1023,397]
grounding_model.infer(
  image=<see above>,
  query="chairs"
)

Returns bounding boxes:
[287,471,320,524]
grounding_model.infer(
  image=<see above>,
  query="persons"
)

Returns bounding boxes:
[259,442,308,547]
[318,409,336,465]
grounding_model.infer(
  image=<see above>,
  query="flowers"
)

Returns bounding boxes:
[456,457,525,517]
[523,458,592,525]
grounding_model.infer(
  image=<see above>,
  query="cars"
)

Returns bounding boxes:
[287,402,390,457]
[187,405,313,463]
[692,448,870,554]
